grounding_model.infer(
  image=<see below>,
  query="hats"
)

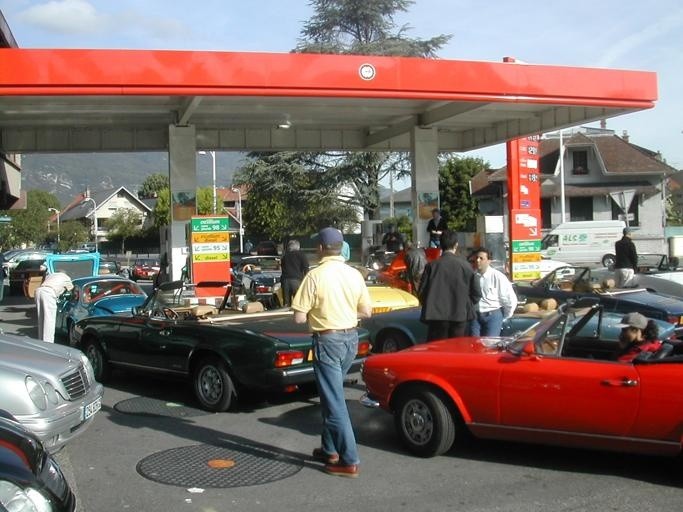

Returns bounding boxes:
[614,312,648,329]
[311,227,344,251]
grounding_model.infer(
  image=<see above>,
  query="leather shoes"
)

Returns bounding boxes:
[313,448,339,464]
[325,464,359,477]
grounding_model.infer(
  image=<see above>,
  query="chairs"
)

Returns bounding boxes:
[540,298,557,310]
[242,301,264,313]
[519,302,539,312]
[191,305,217,315]
[632,343,674,363]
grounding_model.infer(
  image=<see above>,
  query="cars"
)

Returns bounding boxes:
[257,240,277,256]
[1,329,105,456]
[0,411,76,512]
[357,232,683,360]
[230,255,287,305]
[271,265,423,327]
[55,273,151,347]
[1,246,163,301]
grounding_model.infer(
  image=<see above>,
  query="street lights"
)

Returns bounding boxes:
[81,198,99,252]
[231,187,245,255]
[47,207,62,244]
[196,150,217,212]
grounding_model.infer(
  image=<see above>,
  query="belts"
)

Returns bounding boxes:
[320,328,356,335]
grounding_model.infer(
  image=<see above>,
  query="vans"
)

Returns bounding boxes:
[535,219,632,267]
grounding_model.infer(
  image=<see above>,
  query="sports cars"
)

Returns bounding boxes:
[360,296,683,458]
[69,280,373,414]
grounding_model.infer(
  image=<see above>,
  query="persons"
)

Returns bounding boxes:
[290,228,374,478]
[337,229,350,261]
[281,240,309,307]
[427,209,448,248]
[34,270,74,343]
[0,253,7,321]
[612,311,662,363]
[244,240,253,254]
[377,232,428,294]
[615,227,638,289]
[418,230,482,343]
[468,247,517,336]
